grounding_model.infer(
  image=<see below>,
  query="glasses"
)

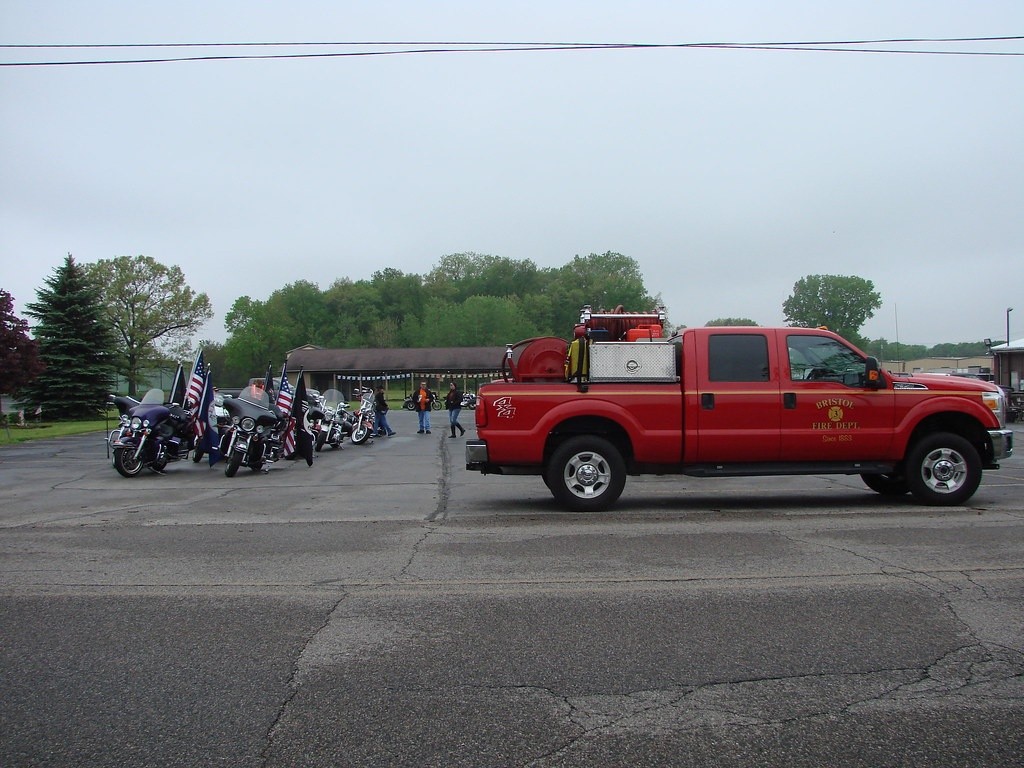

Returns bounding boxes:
[450,384,454,386]
[421,384,426,386]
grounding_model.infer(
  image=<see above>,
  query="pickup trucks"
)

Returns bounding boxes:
[464,323,1013,509]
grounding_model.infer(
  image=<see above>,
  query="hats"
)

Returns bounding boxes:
[377,385,384,389]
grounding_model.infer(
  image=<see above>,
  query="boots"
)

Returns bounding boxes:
[448,425,456,438]
[456,423,465,437]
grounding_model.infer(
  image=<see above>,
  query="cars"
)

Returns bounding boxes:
[443,392,477,410]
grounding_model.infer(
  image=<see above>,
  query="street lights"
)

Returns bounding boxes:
[1006,306,1013,347]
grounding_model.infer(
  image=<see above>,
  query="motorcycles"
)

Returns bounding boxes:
[103,384,378,478]
[403,389,442,411]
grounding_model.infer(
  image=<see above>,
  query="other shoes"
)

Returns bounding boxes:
[417,430,424,433]
[388,431,396,436]
[375,434,382,437]
[426,430,431,434]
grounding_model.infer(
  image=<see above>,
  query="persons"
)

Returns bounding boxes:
[446,382,465,438]
[413,380,434,433]
[373,385,396,436]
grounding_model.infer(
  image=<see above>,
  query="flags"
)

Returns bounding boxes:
[277,371,294,456]
[169,365,185,407]
[290,370,314,466]
[265,367,275,403]
[199,369,220,468]
[186,351,206,441]
